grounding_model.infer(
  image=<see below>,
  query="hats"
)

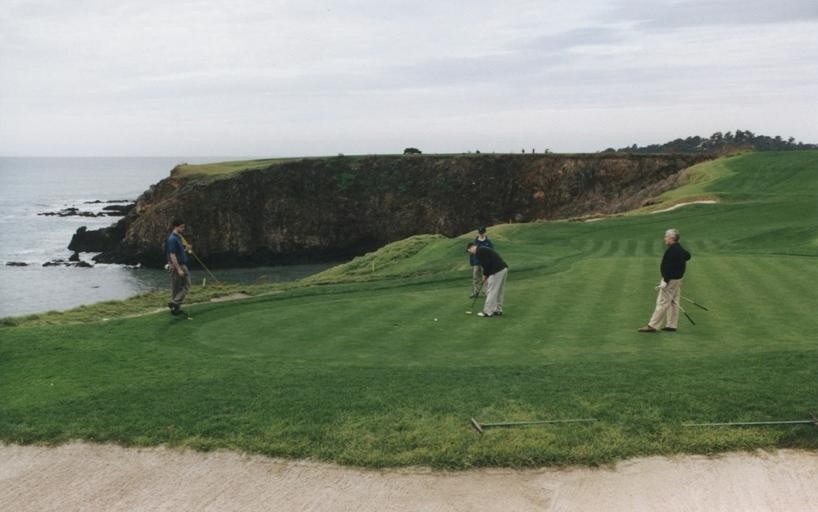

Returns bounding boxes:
[479,226,485,233]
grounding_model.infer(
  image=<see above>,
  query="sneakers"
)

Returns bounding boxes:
[470,290,477,298]
[168,302,179,315]
[477,312,502,317]
[638,325,677,331]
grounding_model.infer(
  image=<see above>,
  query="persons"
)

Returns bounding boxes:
[469,225,493,298]
[466,242,508,317]
[638,228,691,332]
[166,218,192,316]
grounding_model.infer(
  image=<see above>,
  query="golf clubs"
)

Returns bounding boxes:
[656,283,708,312]
[655,285,696,326]
[464,275,488,314]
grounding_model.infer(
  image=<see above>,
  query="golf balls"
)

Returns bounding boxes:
[434,317,438,322]
